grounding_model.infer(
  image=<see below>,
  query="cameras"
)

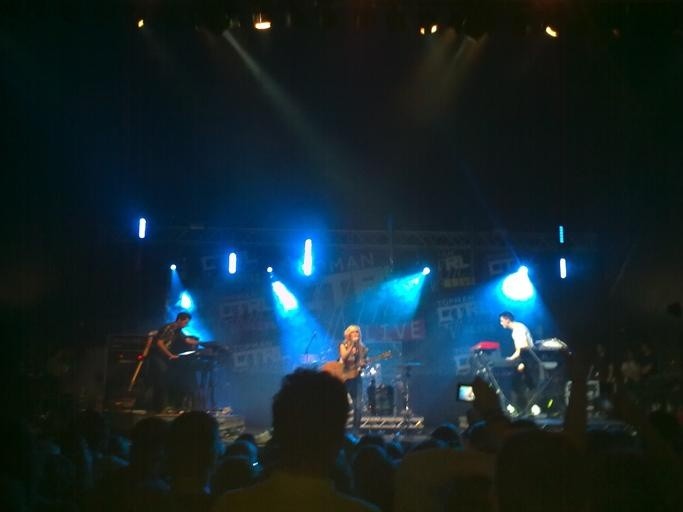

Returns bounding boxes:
[456,384,477,403]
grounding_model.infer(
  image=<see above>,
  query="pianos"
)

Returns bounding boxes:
[169,349,225,369]
[491,360,521,377]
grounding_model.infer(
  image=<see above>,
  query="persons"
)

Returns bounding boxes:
[586,336,656,420]
[339,325,368,441]
[153,312,204,412]
[499,313,536,413]
[1,367,682,511]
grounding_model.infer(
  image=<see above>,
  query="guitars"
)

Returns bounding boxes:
[320,350,391,383]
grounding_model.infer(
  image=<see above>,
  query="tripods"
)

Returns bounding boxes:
[395,369,420,418]
